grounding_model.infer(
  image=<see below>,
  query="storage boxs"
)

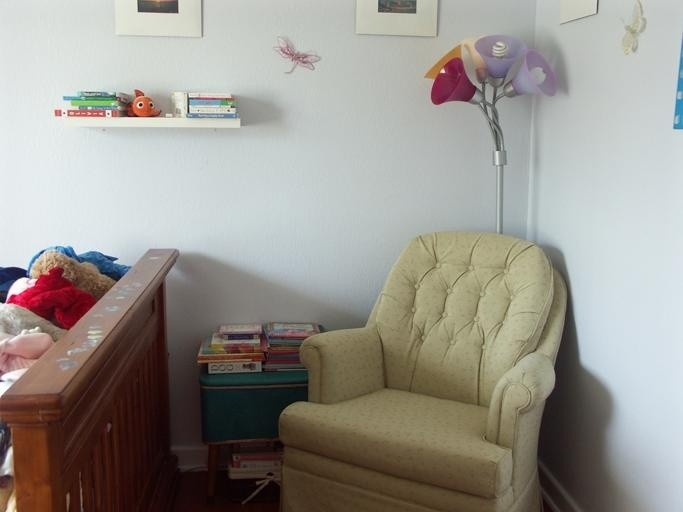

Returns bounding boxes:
[200,372,309,444]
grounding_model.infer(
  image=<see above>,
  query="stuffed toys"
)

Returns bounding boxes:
[29,250,118,302]
[128,89,162,117]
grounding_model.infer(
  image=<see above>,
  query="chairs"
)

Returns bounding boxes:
[278,230,569,512]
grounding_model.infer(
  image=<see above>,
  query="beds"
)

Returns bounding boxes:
[0,249,179,512]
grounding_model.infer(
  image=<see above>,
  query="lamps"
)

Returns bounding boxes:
[424,34,557,235]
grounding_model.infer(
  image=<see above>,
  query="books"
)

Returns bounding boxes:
[186,93,241,119]
[197,321,265,374]
[224,451,282,479]
[52,90,132,117]
[262,321,323,373]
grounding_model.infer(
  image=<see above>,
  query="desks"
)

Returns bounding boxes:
[206,439,281,507]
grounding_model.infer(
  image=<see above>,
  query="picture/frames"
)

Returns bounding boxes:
[356,0,438,37]
[114,0,202,36]
